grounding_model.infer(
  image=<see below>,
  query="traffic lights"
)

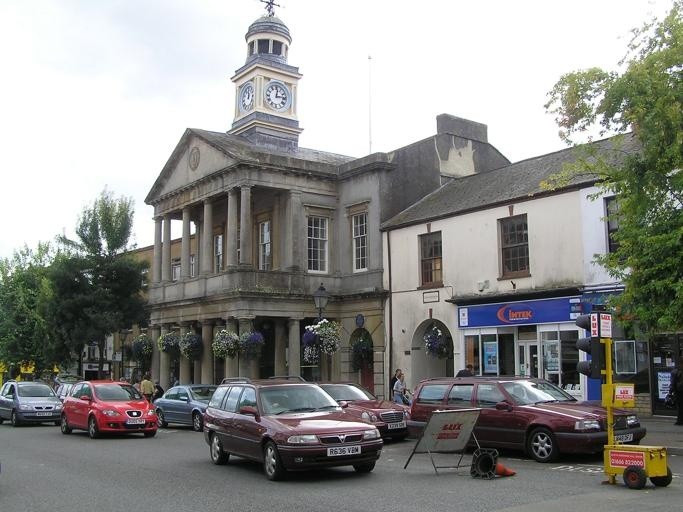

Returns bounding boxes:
[575,310,612,379]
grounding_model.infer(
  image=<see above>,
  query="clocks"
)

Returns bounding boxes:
[264,79,291,112]
[237,81,256,113]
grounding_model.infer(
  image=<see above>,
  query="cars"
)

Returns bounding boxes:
[406,375,647,464]
[309,381,412,445]
[0,372,220,440]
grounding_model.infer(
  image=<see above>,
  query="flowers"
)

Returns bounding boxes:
[124,327,266,360]
[423,325,449,360]
[301,318,373,373]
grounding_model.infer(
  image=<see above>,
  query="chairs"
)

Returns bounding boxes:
[513,388,531,404]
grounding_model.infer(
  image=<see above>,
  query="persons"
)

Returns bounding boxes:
[120,375,163,402]
[391,369,401,397]
[393,373,407,404]
[455,365,474,377]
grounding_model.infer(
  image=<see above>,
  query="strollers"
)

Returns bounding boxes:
[400,387,415,407]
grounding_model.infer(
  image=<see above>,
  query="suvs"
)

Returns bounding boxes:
[202,376,384,482]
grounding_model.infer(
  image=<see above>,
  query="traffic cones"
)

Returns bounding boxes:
[470,447,516,481]
